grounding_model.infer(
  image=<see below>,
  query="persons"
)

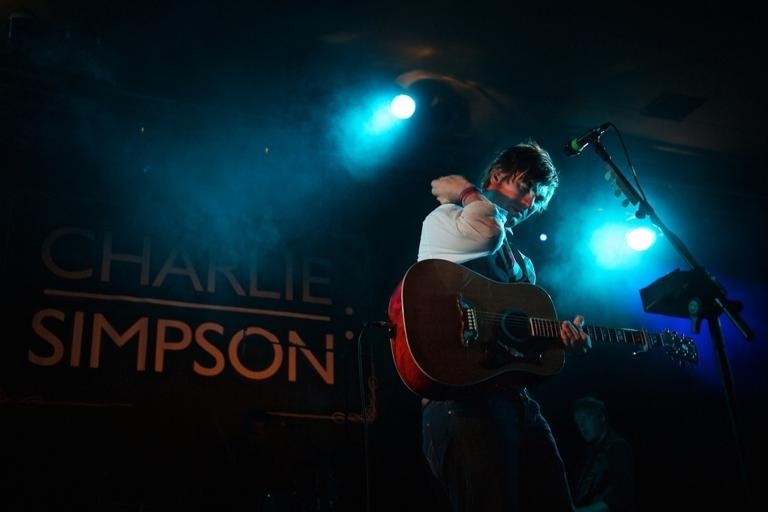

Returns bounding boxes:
[555,396,625,512]
[417,138,594,507]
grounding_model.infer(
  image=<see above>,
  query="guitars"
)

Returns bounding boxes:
[389,259,698,401]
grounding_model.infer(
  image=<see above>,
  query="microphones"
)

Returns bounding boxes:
[562,122,611,157]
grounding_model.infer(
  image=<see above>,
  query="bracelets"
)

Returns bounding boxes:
[459,185,482,206]
